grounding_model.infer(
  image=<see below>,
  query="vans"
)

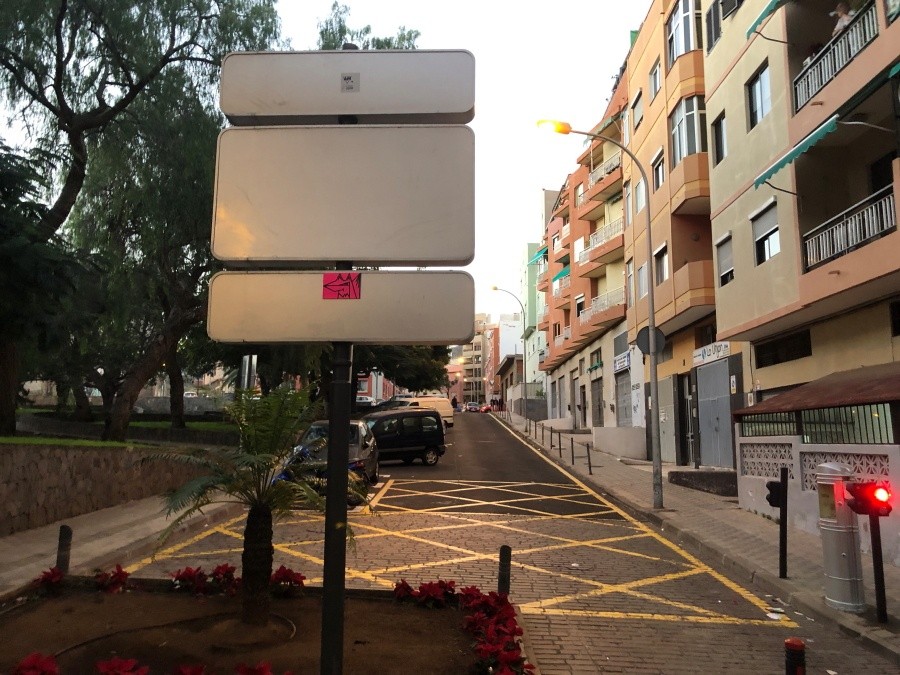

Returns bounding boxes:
[363,409,447,466]
[389,398,454,428]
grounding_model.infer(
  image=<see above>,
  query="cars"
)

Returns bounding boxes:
[462,401,496,414]
[294,417,382,480]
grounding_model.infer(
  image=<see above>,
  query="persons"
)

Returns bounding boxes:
[831,2,854,36]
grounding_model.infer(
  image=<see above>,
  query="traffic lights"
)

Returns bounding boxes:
[870,486,891,516]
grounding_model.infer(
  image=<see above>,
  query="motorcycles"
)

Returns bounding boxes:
[269,448,370,504]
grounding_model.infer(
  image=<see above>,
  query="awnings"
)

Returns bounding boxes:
[551,263,571,289]
[752,62,899,210]
[744,0,836,55]
[527,247,551,273]
[589,113,623,138]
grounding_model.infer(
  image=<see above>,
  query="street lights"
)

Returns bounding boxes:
[492,285,530,432]
[535,118,666,509]
